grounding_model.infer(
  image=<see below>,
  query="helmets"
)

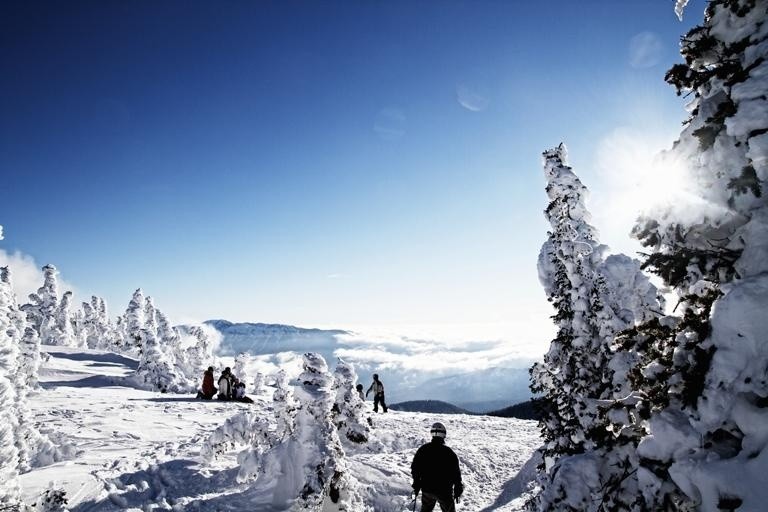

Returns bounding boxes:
[430,422,447,440]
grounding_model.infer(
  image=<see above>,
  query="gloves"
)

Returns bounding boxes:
[412,482,421,498]
[453,485,463,504]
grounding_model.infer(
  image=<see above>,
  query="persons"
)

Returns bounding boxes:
[196,367,253,403]
[411,423,463,512]
[356,384,366,402]
[366,374,387,413]
